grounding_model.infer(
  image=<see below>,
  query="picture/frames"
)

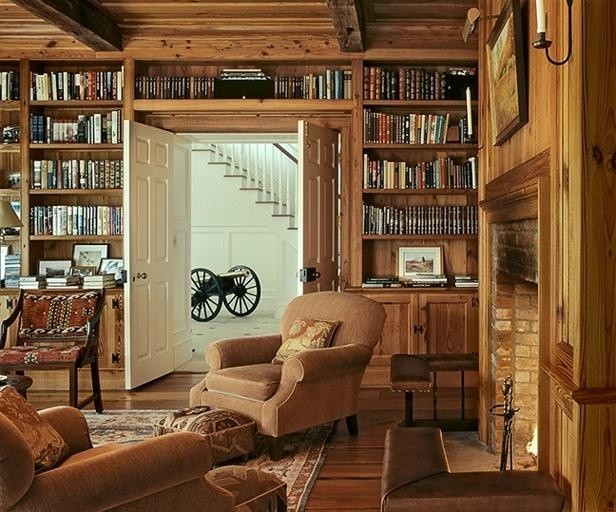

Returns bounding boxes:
[485,0,529,147]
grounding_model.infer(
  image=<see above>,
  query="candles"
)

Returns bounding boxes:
[535,0,546,33]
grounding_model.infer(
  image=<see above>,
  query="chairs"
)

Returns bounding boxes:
[1,286,106,414]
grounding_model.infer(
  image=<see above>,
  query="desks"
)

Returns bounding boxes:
[0,374,32,400]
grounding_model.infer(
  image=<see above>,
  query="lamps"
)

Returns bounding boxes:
[461,8,481,45]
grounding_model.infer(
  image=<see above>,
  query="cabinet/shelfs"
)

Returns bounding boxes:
[0,294,124,372]
[345,288,480,387]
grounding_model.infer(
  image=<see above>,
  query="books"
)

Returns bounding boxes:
[363,64,479,288]
[0,65,124,290]
[133,66,353,99]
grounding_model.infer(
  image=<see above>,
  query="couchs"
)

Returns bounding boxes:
[1,406,289,512]
[151,291,387,461]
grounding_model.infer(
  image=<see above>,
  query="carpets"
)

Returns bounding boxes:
[78,409,340,512]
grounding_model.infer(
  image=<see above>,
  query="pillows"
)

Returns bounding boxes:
[271,315,340,365]
[0,384,70,475]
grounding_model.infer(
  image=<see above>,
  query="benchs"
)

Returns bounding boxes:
[389,352,479,432]
[379,427,566,512]
